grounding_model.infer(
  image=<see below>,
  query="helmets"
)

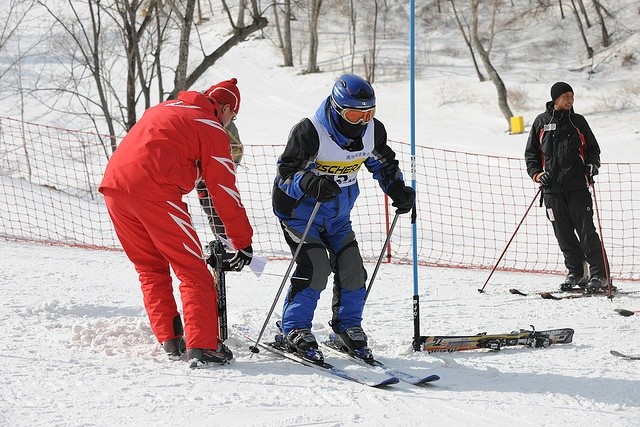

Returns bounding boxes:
[330,73,376,140]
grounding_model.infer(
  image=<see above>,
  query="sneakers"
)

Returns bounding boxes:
[286,329,319,349]
[560,273,589,289]
[340,326,369,347]
[163,336,186,361]
[186,337,234,369]
[586,275,610,292]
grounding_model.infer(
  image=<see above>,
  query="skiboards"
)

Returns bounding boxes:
[232,320,440,387]
[419,324,574,354]
[509,288,639,300]
[610,307,640,360]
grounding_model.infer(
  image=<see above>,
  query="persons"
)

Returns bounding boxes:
[196,120,244,242]
[524,81,610,292]
[98,77,253,366]
[272,73,415,362]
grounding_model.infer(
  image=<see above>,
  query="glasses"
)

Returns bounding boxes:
[231,109,237,120]
[330,94,376,126]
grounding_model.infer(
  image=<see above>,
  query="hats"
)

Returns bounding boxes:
[551,82,574,100]
[203,77,241,114]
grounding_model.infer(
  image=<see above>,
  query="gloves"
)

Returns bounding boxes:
[291,171,342,208]
[586,160,599,178]
[385,180,415,214]
[229,245,253,271]
[533,171,553,187]
